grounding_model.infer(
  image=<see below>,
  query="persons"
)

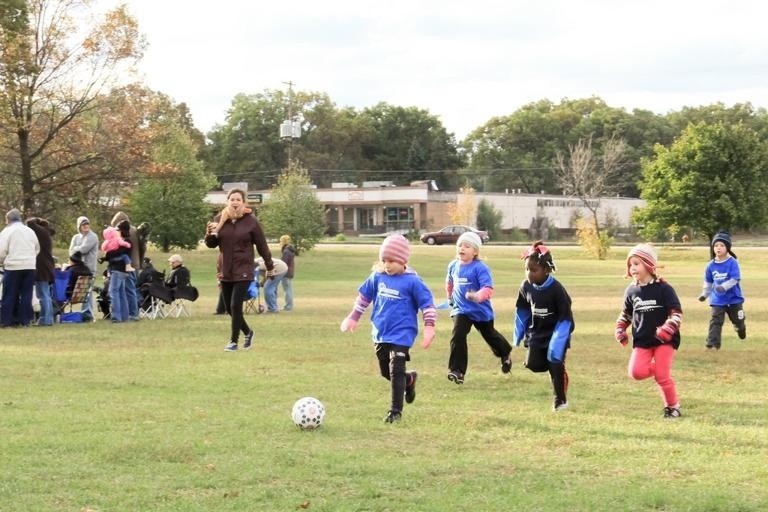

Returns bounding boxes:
[436,233,513,385]
[515,241,575,411]
[340,233,437,424]
[0,207,298,327]
[205,189,275,351]
[698,230,746,352]
[615,245,683,418]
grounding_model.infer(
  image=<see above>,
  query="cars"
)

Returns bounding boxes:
[387,210,396,219]
[417,222,491,247]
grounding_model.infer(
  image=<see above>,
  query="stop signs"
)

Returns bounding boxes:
[399,210,407,216]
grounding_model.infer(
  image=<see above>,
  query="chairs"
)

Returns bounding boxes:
[51,274,97,321]
[245,264,261,314]
[156,268,198,318]
[139,268,166,319]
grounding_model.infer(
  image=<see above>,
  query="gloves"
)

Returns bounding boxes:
[421,326,436,350]
[340,315,359,334]
[465,291,480,303]
[266,269,276,281]
[715,285,726,295]
[614,328,629,346]
[655,324,677,344]
[699,296,705,302]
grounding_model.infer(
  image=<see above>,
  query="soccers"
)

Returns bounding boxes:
[292,397,326,431]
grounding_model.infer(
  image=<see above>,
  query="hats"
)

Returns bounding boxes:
[380,233,410,264]
[168,254,183,263]
[625,242,657,277]
[6,208,21,220]
[711,230,732,250]
[456,231,482,249]
[280,235,290,245]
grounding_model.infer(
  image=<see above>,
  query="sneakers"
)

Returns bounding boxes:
[243,330,256,350]
[551,396,568,411]
[405,370,418,404]
[501,351,512,375]
[384,408,404,425]
[737,329,746,340]
[661,406,682,421]
[447,370,465,385]
[224,342,240,351]
